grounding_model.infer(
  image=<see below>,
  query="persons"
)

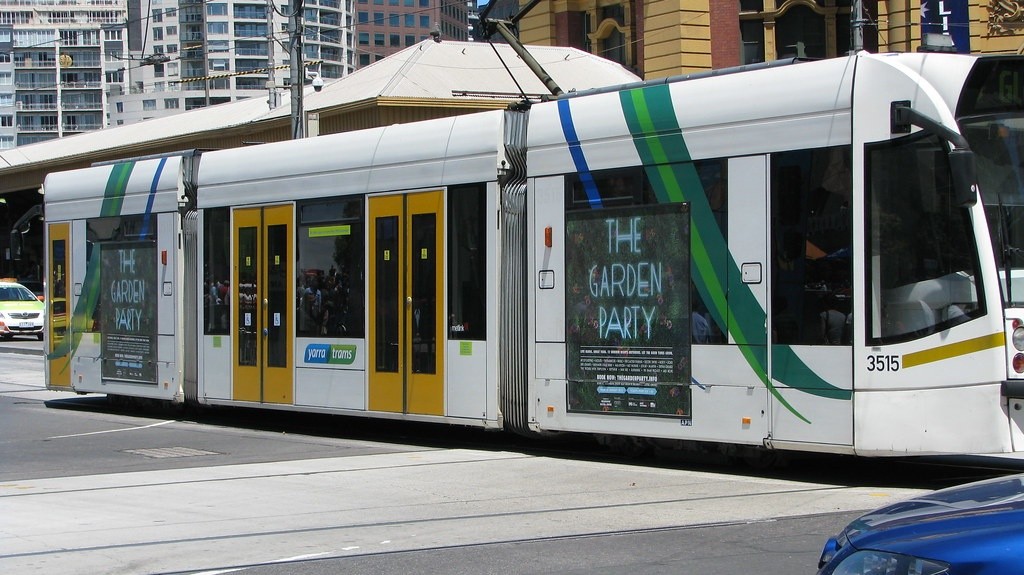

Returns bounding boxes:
[818,295,847,345]
[847,312,854,344]
[412,293,433,374]
[947,303,974,325]
[204,267,352,336]
[691,298,712,345]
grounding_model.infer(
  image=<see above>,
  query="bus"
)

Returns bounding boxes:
[35,1,1024,478]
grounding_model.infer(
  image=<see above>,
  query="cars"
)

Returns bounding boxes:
[817,473,1024,575]
[52,298,67,335]
[0,278,47,342]
